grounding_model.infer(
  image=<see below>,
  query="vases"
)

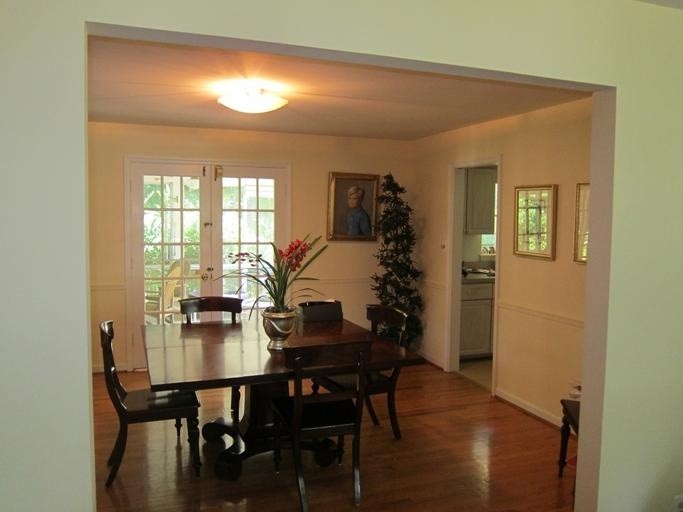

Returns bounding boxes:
[263,309,296,341]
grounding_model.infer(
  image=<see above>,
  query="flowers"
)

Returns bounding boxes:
[209,233,328,310]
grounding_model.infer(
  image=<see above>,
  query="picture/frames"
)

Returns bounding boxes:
[573,183,590,265]
[326,171,380,242]
[511,184,558,261]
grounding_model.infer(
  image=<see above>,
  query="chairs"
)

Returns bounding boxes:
[313,304,410,439]
[144,260,189,325]
[100,321,202,487]
[178,296,245,424]
[269,342,363,511]
[301,300,344,321]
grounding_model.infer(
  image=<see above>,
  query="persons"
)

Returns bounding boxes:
[344,185,372,235]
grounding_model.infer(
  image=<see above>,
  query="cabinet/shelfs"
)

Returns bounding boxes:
[460,282,496,360]
[464,167,495,236]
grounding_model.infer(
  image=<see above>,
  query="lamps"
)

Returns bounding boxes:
[218,92,289,114]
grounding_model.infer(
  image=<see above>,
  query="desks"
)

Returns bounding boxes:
[141,317,423,475]
[559,399,579,479]
